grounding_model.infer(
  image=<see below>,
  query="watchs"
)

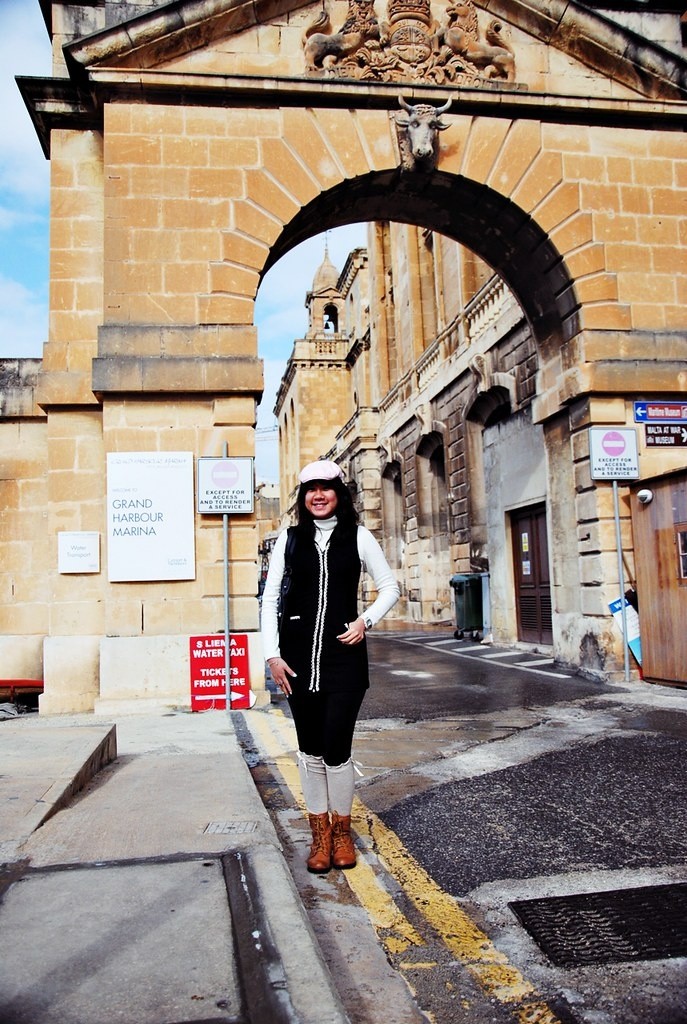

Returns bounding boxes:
[362,615,372,631]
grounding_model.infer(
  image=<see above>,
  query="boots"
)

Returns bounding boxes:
[308,811,357,874]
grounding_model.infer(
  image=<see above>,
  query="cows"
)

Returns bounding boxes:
[393,93,454,162]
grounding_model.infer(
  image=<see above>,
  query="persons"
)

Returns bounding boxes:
[260,459,403,874]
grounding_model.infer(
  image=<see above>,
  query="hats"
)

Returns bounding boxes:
[299,460,344,483]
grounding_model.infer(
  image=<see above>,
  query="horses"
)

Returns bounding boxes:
[433,0,517,84]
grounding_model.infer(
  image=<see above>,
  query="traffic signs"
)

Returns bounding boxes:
[645,424,687,449]
[189,635,250,711]
[632,400,687,424]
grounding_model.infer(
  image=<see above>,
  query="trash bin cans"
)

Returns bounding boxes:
[448,572,484,644]
